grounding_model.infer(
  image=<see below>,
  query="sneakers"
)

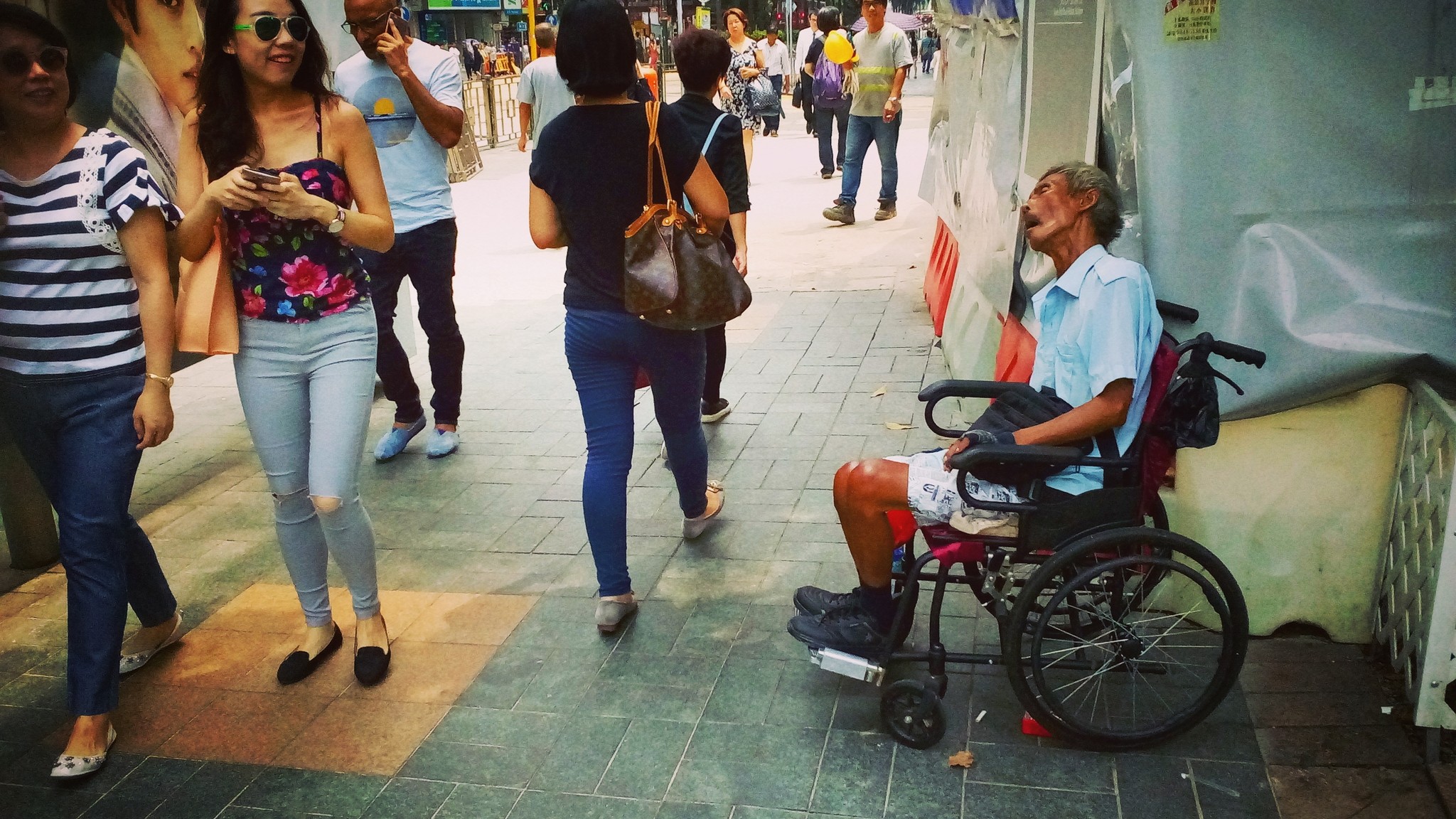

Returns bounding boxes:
[277,621,343,685]
[373,411,427,462]
[104,608,194,675]
[351,608,392,685]
[681,478,724,541]
[763,125,770,136]
[792,585,880,619]
[428,422,461,459]
[874,200,897,220]
[822,204,856,224]
[821,172,832,179]
[836,164,844,172]
[786,605,890,657]
[594,588,640,634]
[51,716,118,779]
[771,128,779,137]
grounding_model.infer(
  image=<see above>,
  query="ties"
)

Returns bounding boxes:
[811,32,816,44]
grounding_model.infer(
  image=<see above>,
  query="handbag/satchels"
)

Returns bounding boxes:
[956,383,1096,492]
[744,89,786,120]
[921,52,929,60]
[624,97,752,332]
[792,79,802,109]
[744,66,779,109]
[172,109,244,358]
[468,52,473,63]
[478,52,484,64]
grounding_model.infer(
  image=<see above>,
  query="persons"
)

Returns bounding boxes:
[655,30,752,424]
[528,0,732,636]
[514,0,942,223]
[1,1,530,778]
[786,163,1162,662]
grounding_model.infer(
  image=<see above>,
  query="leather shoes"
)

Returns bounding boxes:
[813,130,818,138]
[806,121,812,134]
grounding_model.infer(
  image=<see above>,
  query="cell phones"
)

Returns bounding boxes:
[385,12,408,40]
[240,166,281,192]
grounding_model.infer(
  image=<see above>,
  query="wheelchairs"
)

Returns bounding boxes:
[808,298,1256,748]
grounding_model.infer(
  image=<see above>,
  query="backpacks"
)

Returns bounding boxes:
[811,33,853,109]
[908,38,918,57]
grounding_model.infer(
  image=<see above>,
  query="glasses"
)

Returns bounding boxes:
[340,9,396,34]
[232,15,312,42]
[1,46,71,78]
[810,18,819,22]
